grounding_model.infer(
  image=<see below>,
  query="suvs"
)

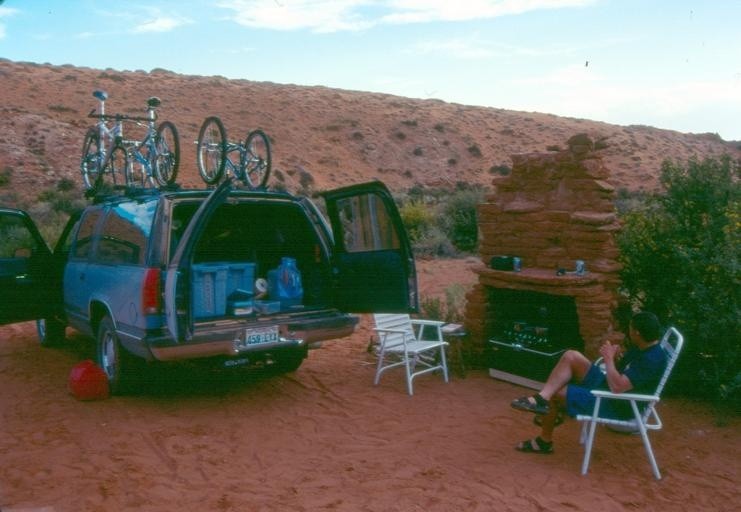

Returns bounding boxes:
[1,179,420,397]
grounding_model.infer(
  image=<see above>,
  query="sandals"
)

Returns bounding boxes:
[515,434,553,454]
[511,393,551,415]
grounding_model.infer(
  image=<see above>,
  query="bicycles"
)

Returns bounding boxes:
[78,91,273,196]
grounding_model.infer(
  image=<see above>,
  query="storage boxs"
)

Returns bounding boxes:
[194,260,255,319]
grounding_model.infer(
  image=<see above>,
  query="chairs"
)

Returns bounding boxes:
[373,313,450,397]
[576,326,683,481]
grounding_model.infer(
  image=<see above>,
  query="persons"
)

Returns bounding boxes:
[510,310,669,454]
